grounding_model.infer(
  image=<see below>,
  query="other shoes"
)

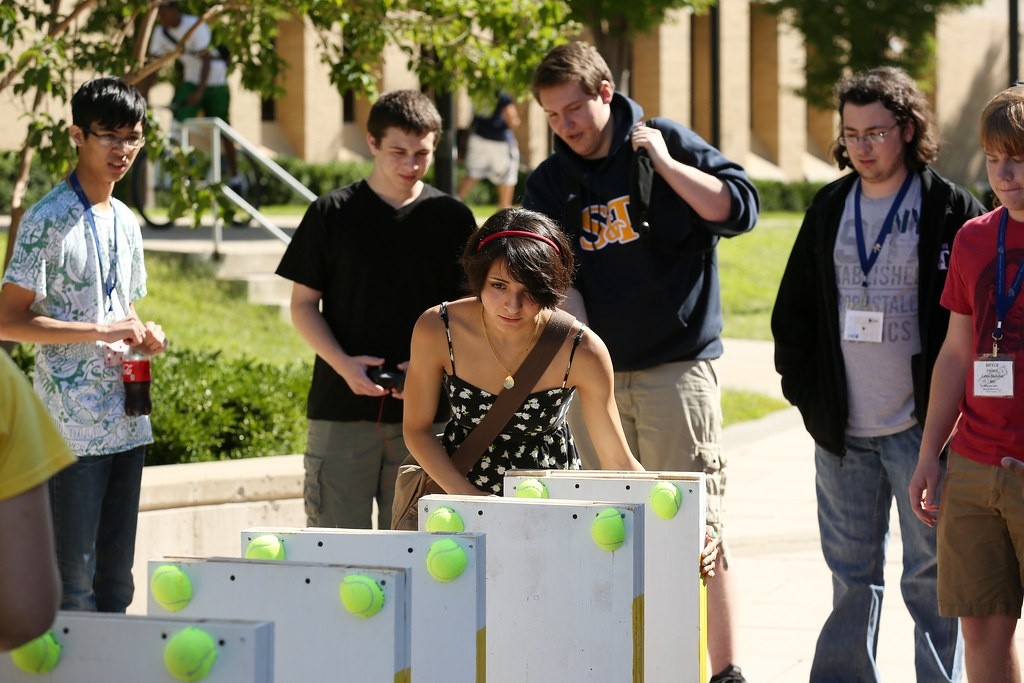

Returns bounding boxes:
[709,664,746,683]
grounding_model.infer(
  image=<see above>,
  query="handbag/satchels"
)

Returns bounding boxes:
[390,451,445,530]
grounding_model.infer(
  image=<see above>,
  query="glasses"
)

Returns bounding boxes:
[837,121,898,147]
[87,129,146,149]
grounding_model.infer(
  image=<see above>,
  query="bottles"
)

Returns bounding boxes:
[123,341,151,417]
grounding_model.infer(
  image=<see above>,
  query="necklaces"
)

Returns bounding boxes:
[481,305,541,390]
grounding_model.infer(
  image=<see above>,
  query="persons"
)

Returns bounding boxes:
[276,90,478,531]
[909,85,1024,683]
[458,86,521,207]
[402,207,717,577]
[0,350,78,653]
[770,68,990,683]
[0,78,168,613]
[527,43,760,683]
